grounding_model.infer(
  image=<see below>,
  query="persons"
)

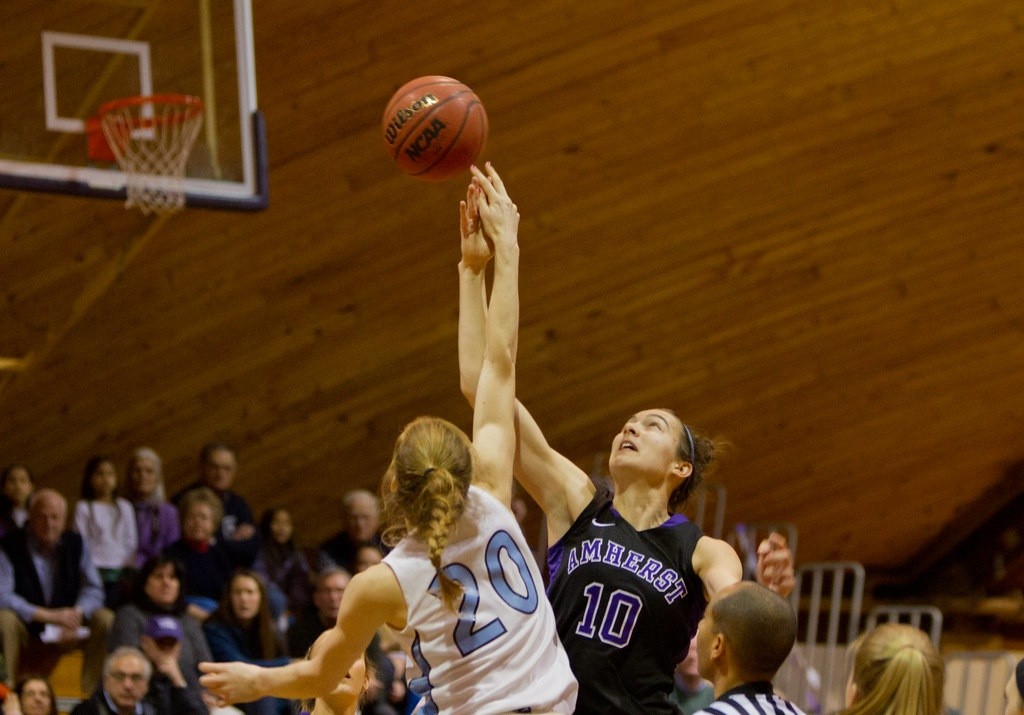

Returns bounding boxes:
[197,161,580,715]
[0,442,421,715]
[457,175,793,715]
[833,623,946,715]
[664,632,714,715]
[690,581,807,715]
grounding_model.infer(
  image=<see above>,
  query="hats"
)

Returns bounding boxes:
[145,614,183,639]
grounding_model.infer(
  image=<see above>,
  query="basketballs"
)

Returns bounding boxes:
[382,74,489,183]
